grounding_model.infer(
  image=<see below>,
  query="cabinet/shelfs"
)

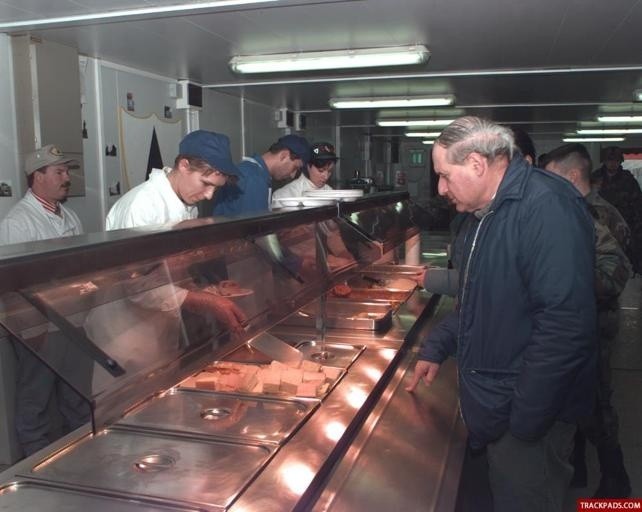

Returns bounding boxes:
[1,185,469,510]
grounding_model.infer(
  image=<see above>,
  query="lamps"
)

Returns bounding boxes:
[330,92,454,112]
[560,90,642,146]
[376,114,460,147]
[225,44,430,78]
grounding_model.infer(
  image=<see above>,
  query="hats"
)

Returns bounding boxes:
[309,141,340,162]
[276,132,314,166]
[22,142,81,177]
[177,128,247,179]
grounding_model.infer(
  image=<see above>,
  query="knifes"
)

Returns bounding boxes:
[240,323,303,370]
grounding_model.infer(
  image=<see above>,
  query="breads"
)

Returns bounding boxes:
[178,360,261,394]
[262,359,330,398]
[217,279,240,295]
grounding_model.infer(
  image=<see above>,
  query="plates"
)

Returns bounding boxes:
[200,286,254,299]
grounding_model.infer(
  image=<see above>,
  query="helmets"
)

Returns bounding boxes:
[599,145,625,164]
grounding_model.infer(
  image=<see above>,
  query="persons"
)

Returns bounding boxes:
[80,129,248,392]
[209,134,312,277]
[271,141,354,274]
[405,114,642,510]
[0,143,90,456]
